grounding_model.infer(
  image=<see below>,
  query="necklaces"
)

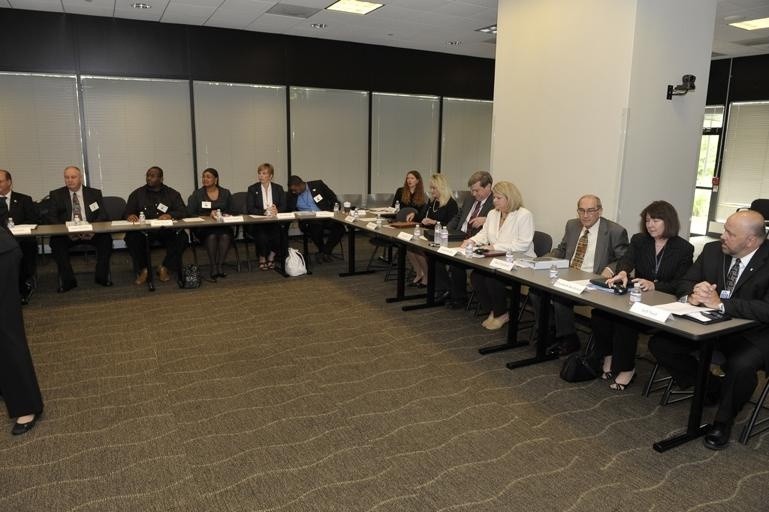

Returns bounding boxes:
[432,199,440,212]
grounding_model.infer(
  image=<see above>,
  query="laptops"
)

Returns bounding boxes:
[590,276,639,288]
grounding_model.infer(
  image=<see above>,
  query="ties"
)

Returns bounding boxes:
[570,230,589,271]
[467,201,481,238]
[725,258,741,295]
[73,193,82,221]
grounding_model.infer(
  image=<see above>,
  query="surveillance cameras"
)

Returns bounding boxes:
[678,74,696,89]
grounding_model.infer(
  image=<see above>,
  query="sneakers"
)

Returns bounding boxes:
[156,264,170,282]
[134,268,148,285]
[315,252,330,264]
[482,311,510,330]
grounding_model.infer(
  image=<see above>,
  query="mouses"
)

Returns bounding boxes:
[475,249,484,254]
[614,287,627,295]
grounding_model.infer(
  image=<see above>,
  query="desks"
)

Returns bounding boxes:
[332,209,755,450]
[8,211,342,291]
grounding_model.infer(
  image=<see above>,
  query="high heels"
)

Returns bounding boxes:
[210,272,227,283]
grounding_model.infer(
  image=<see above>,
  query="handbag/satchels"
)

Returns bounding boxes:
[178,264,201,289]
[560,356,599,383]
[285,247,307,277]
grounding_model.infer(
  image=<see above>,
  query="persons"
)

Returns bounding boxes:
[0,228,43,436]
[122,166,186,285]
[245,163,287,271]
[49,166,113,293]
[0,170,36,294]
[287,175,345,263]
[393,170,430,266]
[433,171,495,310]
[538,195,629,359]
[460,181,537,330]
[187,168,231,277]
[649,211,768,448]
[406,174,459,289]
[602,200,695,392]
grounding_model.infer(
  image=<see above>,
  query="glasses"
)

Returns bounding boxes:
[577,209,599,214]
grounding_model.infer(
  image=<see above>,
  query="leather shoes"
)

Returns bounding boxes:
[12,415,36,435]
[549,339,580,356]
[408,277,427,289]
[95,279,112,287]
[703,421,732,450]
[57,285,77,292]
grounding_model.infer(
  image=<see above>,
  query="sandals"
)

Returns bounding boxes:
[259,261,276,271]
[600,356,636,391]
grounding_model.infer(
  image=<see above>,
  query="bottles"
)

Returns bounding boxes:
[413,224,420,240]
[628,283,641,304]
[334,203,339,216]
[353,209,358,223]
[74,215,80,228]
[344,202,351,216]
[439,226,449,249]
[7,217,14,232]
[505,248,513,263]
[395,201,399,212]
[216,209,221,223]
[550,263,558,282]
[434,222,441,244]
[139,212,145,224]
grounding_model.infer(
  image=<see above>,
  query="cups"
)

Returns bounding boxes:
[465,240,473,259]
[377,213,381,223]
